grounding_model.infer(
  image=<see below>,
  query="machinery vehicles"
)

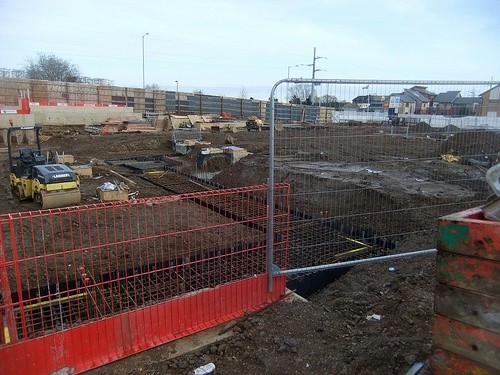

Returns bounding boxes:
[7,118,82,210]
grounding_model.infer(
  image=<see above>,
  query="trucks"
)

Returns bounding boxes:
[246,119,263,132]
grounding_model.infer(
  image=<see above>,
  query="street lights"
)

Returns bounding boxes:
[175,80,178,91]
[142,32,149,88]
[286,65,298,103]
